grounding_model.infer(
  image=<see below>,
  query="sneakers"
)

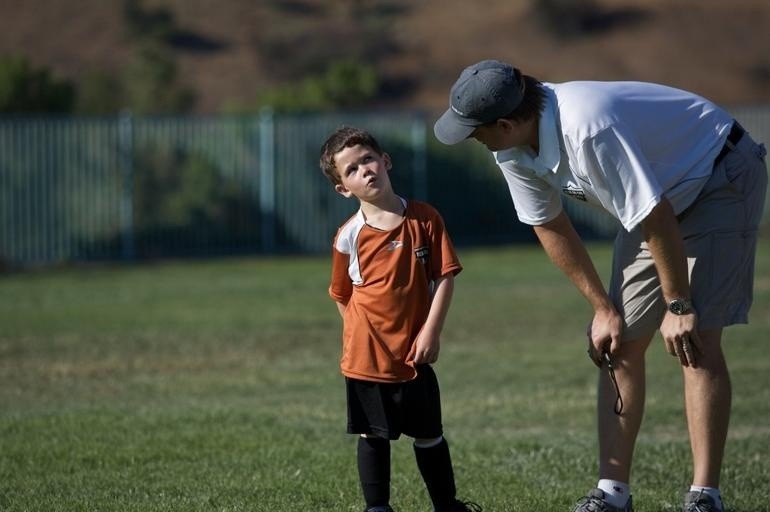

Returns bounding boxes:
[682,488,725,512]
[570,488,633,511]
[434,499,482,512]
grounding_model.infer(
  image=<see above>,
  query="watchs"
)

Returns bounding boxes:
[666,298,692,316]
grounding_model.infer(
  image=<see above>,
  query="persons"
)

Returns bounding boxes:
[433,58,766,512]
[319,125,477,512]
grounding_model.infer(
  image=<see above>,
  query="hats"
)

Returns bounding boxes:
[433,58,527,147]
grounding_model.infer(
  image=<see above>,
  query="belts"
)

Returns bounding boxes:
[713,119,745,169]
